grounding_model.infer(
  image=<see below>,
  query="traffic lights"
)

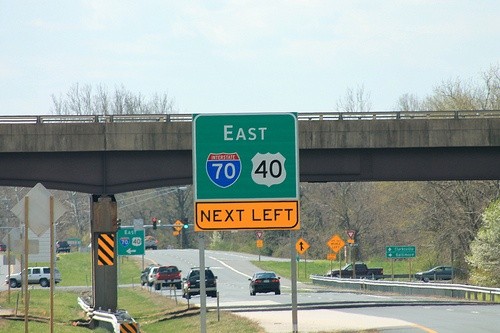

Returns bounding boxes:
[152,217,157,230]
[117,218,122,232]
[183,217,189,230]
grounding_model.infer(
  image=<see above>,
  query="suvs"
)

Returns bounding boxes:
[182,266,218,300]
[6,266,62,287]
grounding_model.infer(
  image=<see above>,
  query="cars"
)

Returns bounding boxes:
[415,266,468,283]
[140,263,182,290]
[248,271,281,296]
[144,235,158,250]
[0,240,6,252]
[56,240,71,253]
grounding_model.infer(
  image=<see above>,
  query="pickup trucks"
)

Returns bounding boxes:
[324,263,384,280]
